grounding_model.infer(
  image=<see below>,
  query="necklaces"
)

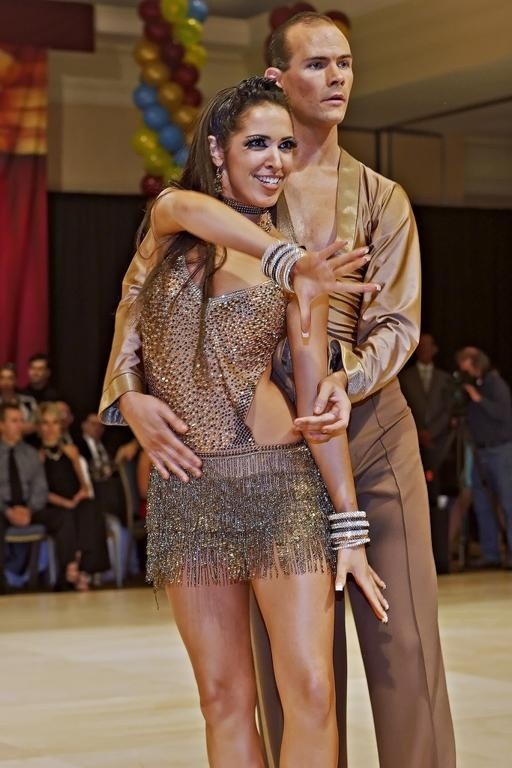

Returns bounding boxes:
[221,198,268,215]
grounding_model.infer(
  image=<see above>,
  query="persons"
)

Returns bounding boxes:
[0,352,152,594]
[398,330,512,574]
[97,12,456,768]
[138,78,389,768]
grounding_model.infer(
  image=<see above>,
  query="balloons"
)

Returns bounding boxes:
[270,2,352,47]
[133,0,208,200]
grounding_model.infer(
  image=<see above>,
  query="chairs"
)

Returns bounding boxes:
[0,460,149,596]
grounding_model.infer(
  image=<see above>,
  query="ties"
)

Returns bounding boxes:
[9,447,22,506]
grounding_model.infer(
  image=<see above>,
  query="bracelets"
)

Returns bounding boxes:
[327,511,371,551]
[261,241,306,294]
